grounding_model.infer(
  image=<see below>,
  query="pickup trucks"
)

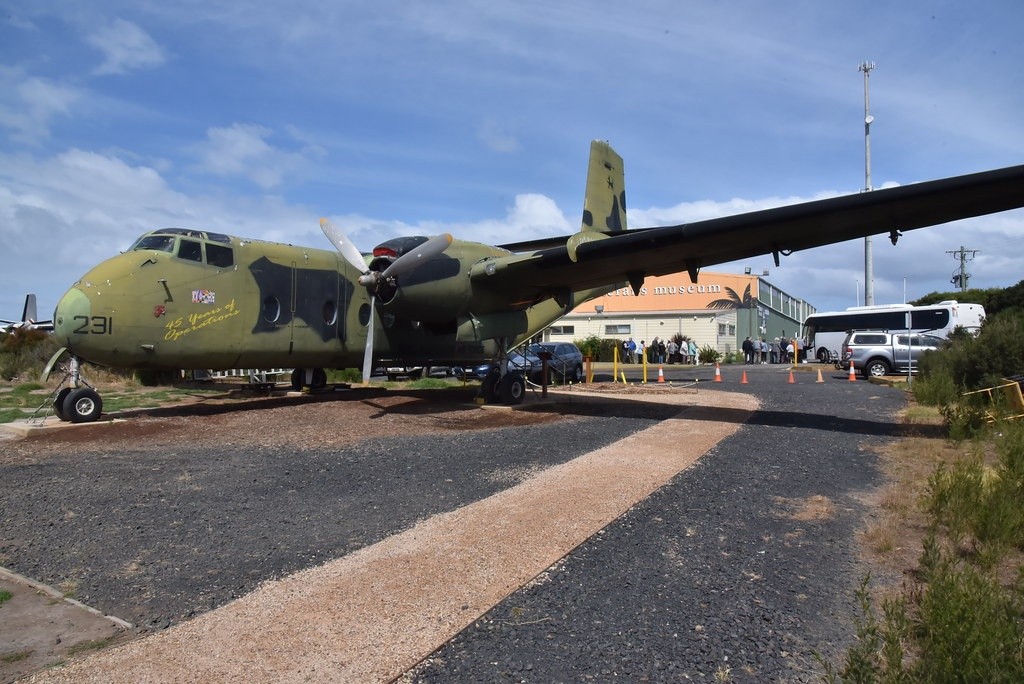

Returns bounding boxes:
[840,330,949,380]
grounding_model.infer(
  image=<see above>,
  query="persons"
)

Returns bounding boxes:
[680,337,698,365]
[658,338,667,365]
[651,337,660,364]
[767,334,814,363]
[620,337,631,364]
[638,340,645,365]
[628,338,636,364]
[667,339,679,365]
[741,336,767,365]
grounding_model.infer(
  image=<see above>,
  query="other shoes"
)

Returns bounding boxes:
[764,362,767,364]
[774,362,779,364]
[761,362,763,364]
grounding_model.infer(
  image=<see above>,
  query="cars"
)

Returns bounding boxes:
[372,366,455,381]
[451,365,500,381]
[508,342,583,385]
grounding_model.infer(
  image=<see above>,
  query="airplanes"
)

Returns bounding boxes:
[0,294,54,334]
[26,139,1024,425]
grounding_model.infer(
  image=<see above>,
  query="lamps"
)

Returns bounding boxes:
[694,316,697,320]
[711,316,714,320]
[595,306,604,313]
[745,267,751,274]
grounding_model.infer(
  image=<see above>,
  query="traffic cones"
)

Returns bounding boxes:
[713,362,723,383]
[848,361,857,382]
[816,369,824,383]
[657,365,666,383]
[787,371,794,383]
[741,371,749,384]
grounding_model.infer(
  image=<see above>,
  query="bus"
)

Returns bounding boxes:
[801,299,987,363]
